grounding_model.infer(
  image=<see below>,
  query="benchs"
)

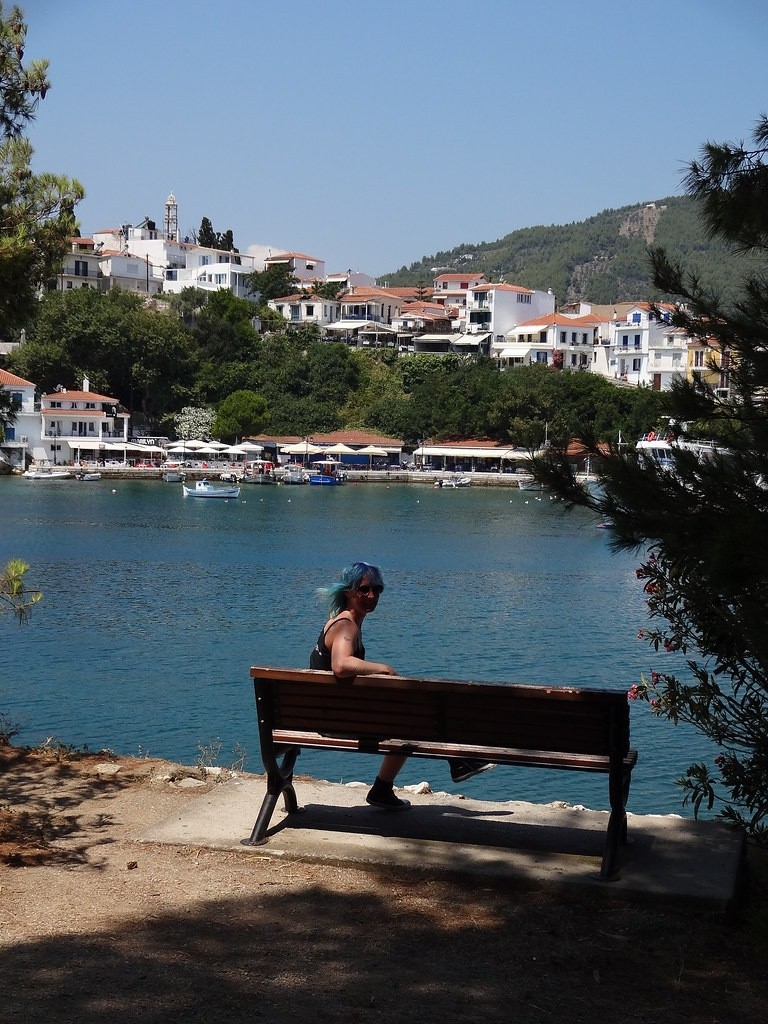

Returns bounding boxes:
[241,667,638,883]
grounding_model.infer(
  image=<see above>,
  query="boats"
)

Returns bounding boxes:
[434,472,472,488]
[182,477,241,498]
[162,471,187,482]
[21,467,73,480]
[74,472,102,481]
[634,433,768,507]
[221,454,349,486]
[518,422,611,495]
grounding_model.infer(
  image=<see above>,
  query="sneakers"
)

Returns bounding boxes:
[366,784,410,811]
[451,760,497,783]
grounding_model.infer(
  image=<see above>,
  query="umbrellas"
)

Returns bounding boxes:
[324,442,358,462]
[280,441,323,464]
[166,439,264,462]
[140,446,164,461]
[356,445,388,468]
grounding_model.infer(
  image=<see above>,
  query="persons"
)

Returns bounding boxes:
[309,562,497,809]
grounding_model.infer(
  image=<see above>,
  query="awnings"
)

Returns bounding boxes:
[450,333,492,345]
[413,448,545,469]
[499,348,530,358]
[507,325,549,335]
[67,441,149,461]
[321,322,367,344]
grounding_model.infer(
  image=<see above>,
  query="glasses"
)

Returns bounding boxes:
[348,585,384,596]
